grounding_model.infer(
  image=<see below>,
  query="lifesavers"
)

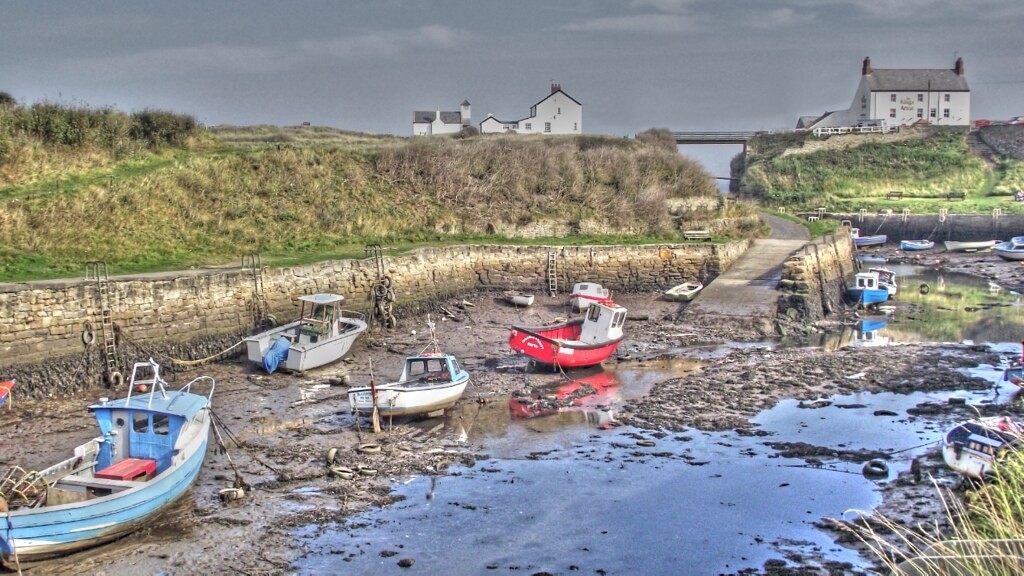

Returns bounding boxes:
[598,299,614,304]
[108,371,123,389]
[862,460,889,477]
[81,328,97,345]
[374,275,397,328]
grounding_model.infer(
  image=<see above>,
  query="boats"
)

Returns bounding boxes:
[941,415,1023,482]
[855,252,887,265]
[902,239,935,250]
[994,364,1024,407]
[509,298,628,367]
[853,235,888,245]
[849,268,899,306]
[664,279,704,302]
[943,240,996,249]
[569,281,610,314]
[992,236,1023,261]
[503,289,536,306]
[0,358,215,569]
[347,320,469,414]
[243,292,368,372]
